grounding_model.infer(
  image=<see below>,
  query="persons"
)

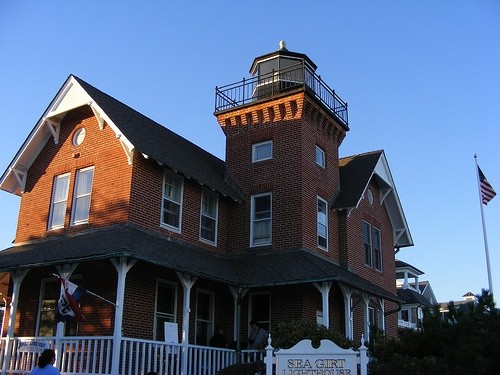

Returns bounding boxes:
[247,320,268,349]
[208,327,228,349]
[30,349,59,375]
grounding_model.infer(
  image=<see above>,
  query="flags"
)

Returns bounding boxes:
[477,164,496,206]
[55,277,84,322]
